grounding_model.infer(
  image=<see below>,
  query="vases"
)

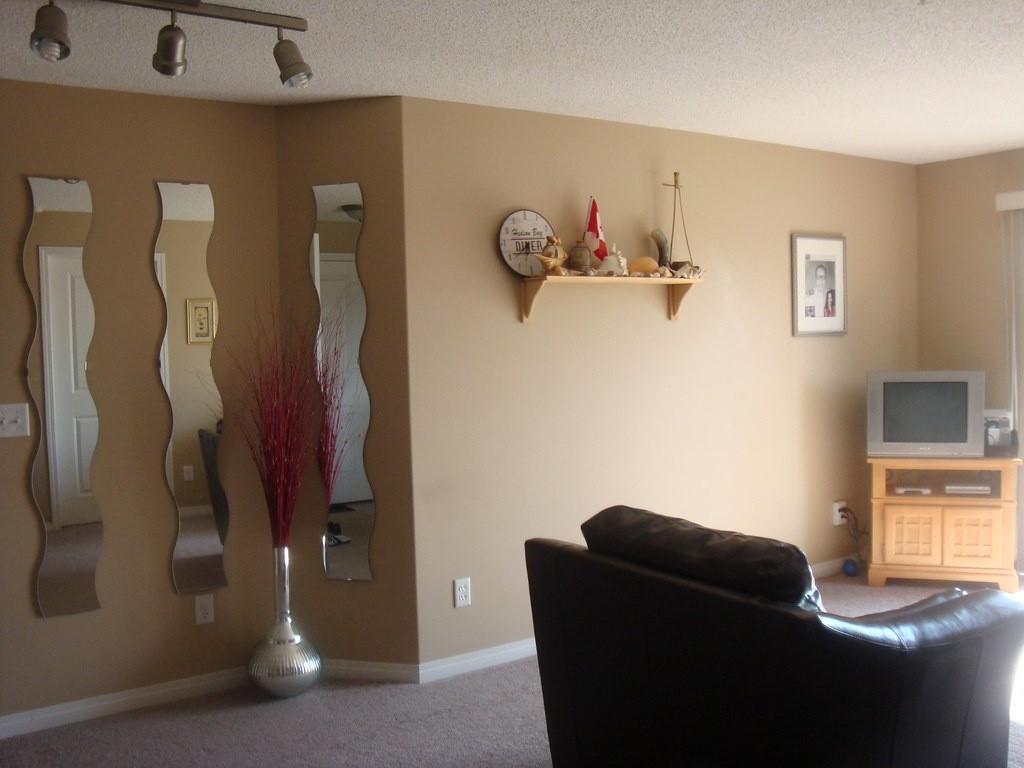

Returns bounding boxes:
[244,547,321,697]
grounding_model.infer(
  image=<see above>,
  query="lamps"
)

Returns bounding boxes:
[273,24,311,91]
[152,9,188,79]
[28,1,70,61]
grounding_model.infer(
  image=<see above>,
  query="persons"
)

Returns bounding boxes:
[824,291,836,317]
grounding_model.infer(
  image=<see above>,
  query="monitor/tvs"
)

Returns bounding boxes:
[866,370,984,459]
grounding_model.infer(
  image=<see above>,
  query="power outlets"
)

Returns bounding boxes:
[453,576,471,607]
[19,175,105,619]
[833,502,849,526]
[0,403,31,438]
[194,594,215,627]
[184,297,216,346]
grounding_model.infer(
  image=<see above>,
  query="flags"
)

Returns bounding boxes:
[586,199,607,261]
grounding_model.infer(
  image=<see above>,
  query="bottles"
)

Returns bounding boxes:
[568,240,591,276]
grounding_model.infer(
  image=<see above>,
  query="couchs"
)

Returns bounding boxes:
[523,500,1023,768]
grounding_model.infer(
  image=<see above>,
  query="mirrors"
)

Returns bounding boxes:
[305,180,382,579]
[152,180,225,595]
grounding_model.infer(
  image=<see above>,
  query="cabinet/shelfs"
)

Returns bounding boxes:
[869,455,1022,595]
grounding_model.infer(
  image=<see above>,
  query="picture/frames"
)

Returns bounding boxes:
[790,235,852,337]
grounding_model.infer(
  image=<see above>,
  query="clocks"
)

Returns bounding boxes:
[499,210,553,276]
